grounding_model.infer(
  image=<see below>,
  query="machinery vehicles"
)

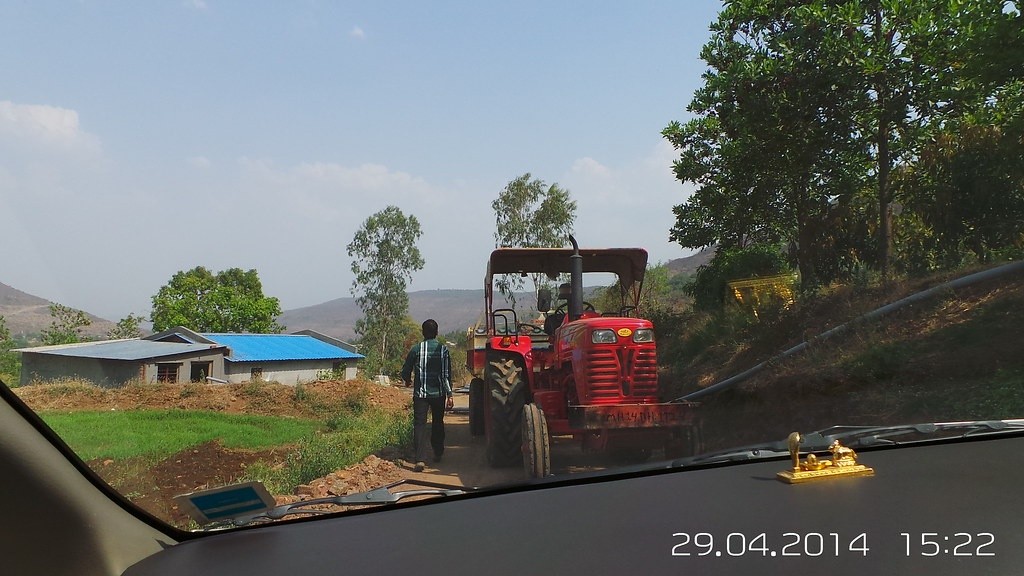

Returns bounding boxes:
[466,235,707,481]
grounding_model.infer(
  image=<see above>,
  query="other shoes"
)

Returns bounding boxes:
[413,461,425,472]
[435,456,441,463]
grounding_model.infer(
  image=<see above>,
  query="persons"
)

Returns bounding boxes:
[402,319,454,472]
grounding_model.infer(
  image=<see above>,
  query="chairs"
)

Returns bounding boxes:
[544,314,566,335]
